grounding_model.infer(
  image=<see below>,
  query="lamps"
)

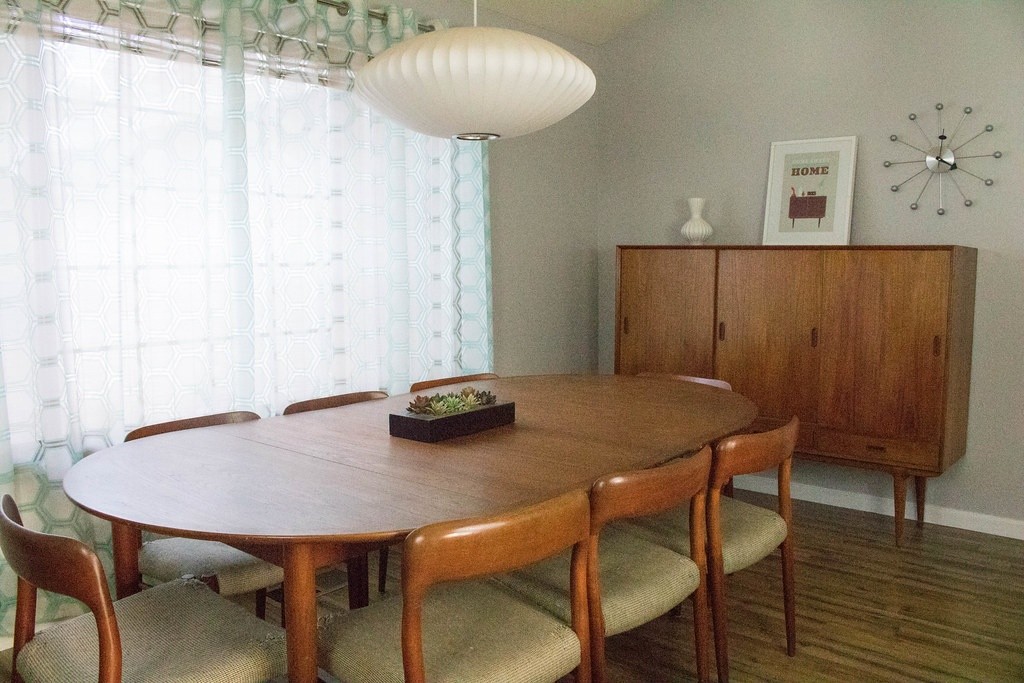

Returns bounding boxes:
[353,0,600,145]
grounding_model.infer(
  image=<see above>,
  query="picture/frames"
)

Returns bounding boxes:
[763,134,858,245]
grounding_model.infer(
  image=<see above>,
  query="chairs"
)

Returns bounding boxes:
[411,369,499,391]
[0,493,292,683]
[320,487,592,683]
[125,409,285,627]
[613,416,805,683]
[510,444,711,683]
[282,388,389,413]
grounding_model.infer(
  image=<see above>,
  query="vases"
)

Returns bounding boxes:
[680,197,712,242]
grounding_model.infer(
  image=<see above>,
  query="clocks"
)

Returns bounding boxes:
[882,102,1003,214]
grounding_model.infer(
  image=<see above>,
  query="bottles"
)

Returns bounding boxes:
[680,198,714,247]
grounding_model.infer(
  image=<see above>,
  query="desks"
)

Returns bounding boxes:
[66,363,762,672]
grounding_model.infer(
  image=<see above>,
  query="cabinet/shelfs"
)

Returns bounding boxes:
[607,238,977,543]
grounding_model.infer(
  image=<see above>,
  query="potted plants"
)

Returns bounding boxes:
[388,386,515,442]
